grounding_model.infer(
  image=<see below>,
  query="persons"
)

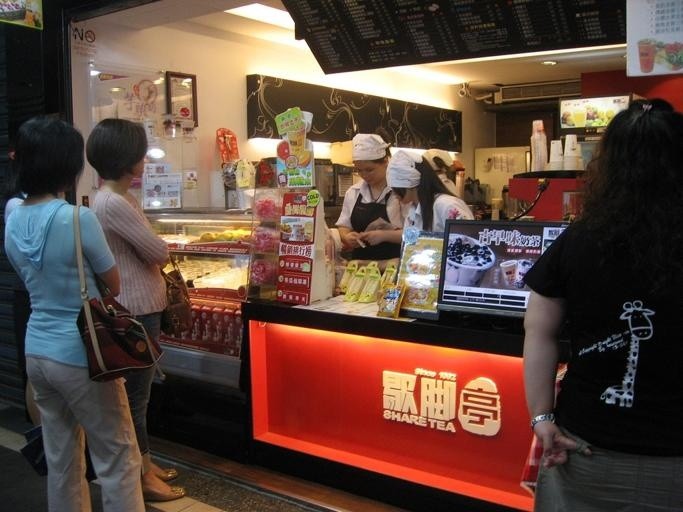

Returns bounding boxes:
[4,112,145,511]
[523,99,683,512]
[86,118,187,500]
[386,151,474,242]
[6,150,62,426]
[335,133,412,261]
[421,148,465,199]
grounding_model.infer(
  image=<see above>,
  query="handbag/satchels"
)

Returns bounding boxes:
[74,204,166,383]
[159,252,193,336]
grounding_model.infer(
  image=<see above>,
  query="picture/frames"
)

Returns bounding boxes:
[165,70,199,129]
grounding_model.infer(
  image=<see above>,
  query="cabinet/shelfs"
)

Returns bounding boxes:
[139,206,337,395]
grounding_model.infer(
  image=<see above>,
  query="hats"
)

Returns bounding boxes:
[386,150,423,188]
[423,148,454,171]
[352,134,388,160]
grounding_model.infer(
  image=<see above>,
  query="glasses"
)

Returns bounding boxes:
[355,164,382,174]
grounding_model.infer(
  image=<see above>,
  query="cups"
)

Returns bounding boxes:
[636,38,658,74]
[286,120,307,161]
[512,259,534,289]
[529,121,547,172]
[565,135,584,170]
[549,140,564,170]
[500,260,518,289]
[445,233,496,287]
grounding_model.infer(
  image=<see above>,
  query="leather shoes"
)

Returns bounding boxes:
[144,485,186,501]
[154,468,178,481]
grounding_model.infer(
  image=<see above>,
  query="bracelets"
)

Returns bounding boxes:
[527,413,560,430]
